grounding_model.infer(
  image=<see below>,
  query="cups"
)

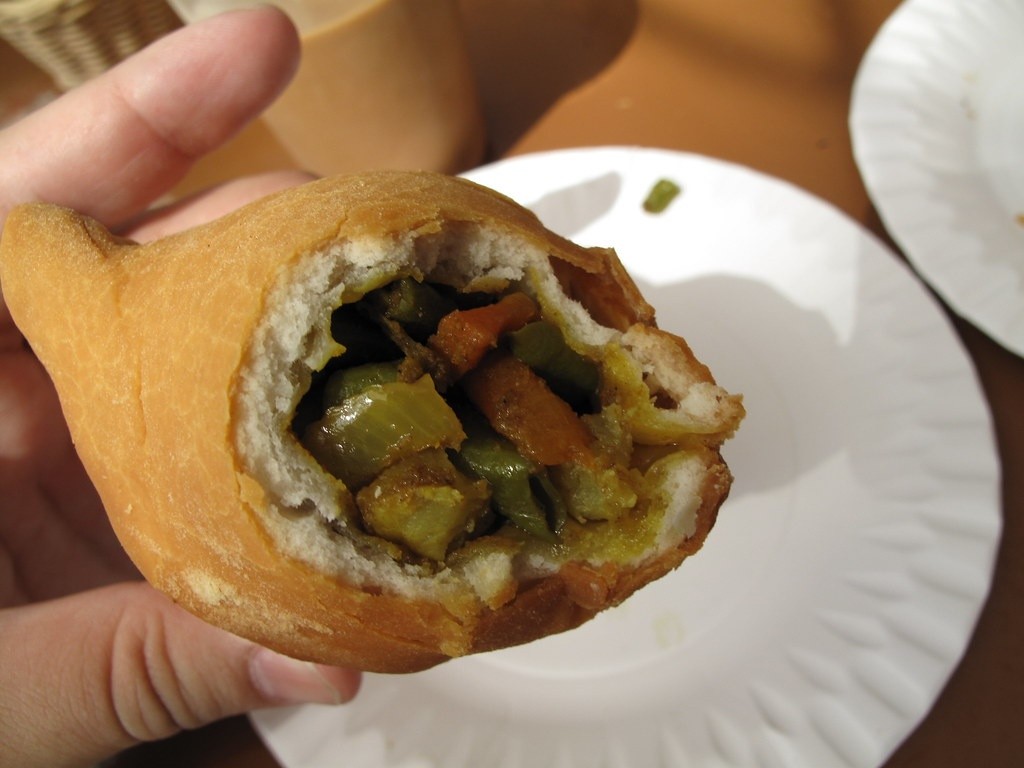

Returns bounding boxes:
[166,0,488,181]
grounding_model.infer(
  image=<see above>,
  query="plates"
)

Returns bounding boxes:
[239,141,1003,768]
[846,1,1024,359]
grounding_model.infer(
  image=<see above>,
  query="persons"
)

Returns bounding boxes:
[2,1,370,768]
[168,1,647,193]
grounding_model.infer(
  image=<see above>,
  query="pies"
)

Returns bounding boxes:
[0,172,745,672]
